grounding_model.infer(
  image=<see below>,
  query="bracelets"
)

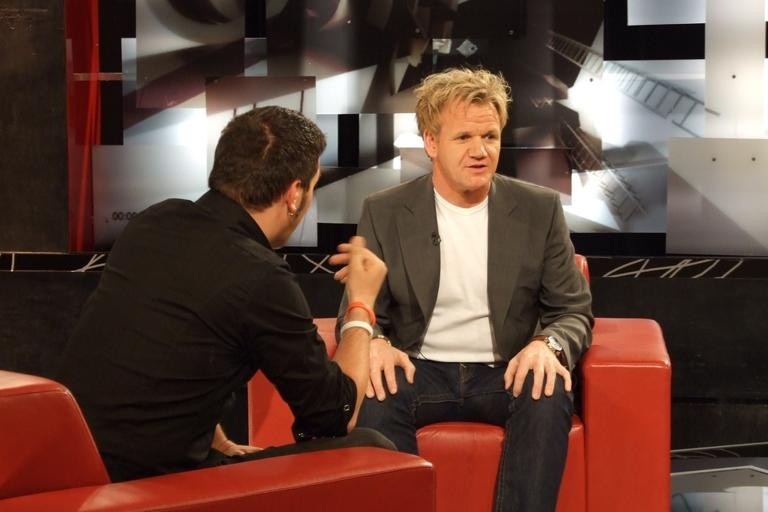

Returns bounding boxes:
[339,302,376,339]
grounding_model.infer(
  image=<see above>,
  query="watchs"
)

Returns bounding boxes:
[527,335,569,370]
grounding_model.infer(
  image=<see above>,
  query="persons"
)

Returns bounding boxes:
[337,69,594,512]
[57,107,398,484]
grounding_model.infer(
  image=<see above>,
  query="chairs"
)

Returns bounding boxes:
[246,253,673,512]
[1,371,438,512]
